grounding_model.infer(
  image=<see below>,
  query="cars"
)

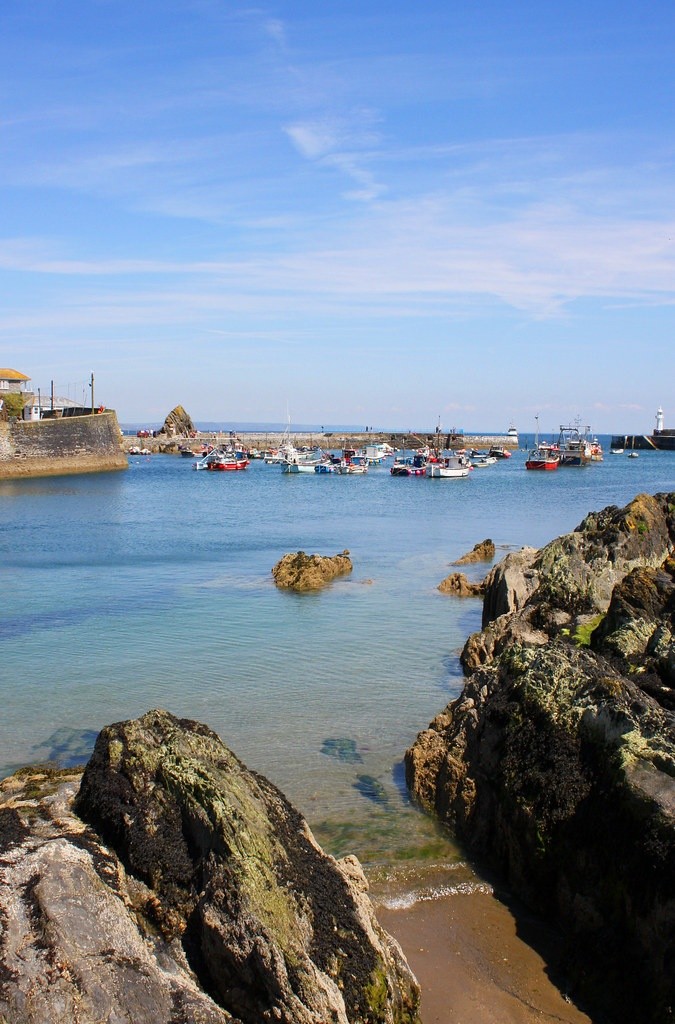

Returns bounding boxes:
[137,430,149,438]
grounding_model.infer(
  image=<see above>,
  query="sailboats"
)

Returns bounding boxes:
[628,436,639,458]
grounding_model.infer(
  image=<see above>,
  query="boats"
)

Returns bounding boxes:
[552,426,604,467]
[129,439,151,455]
[180,438,511,478]
[612,448,622,454]
[525,442,560,470]
